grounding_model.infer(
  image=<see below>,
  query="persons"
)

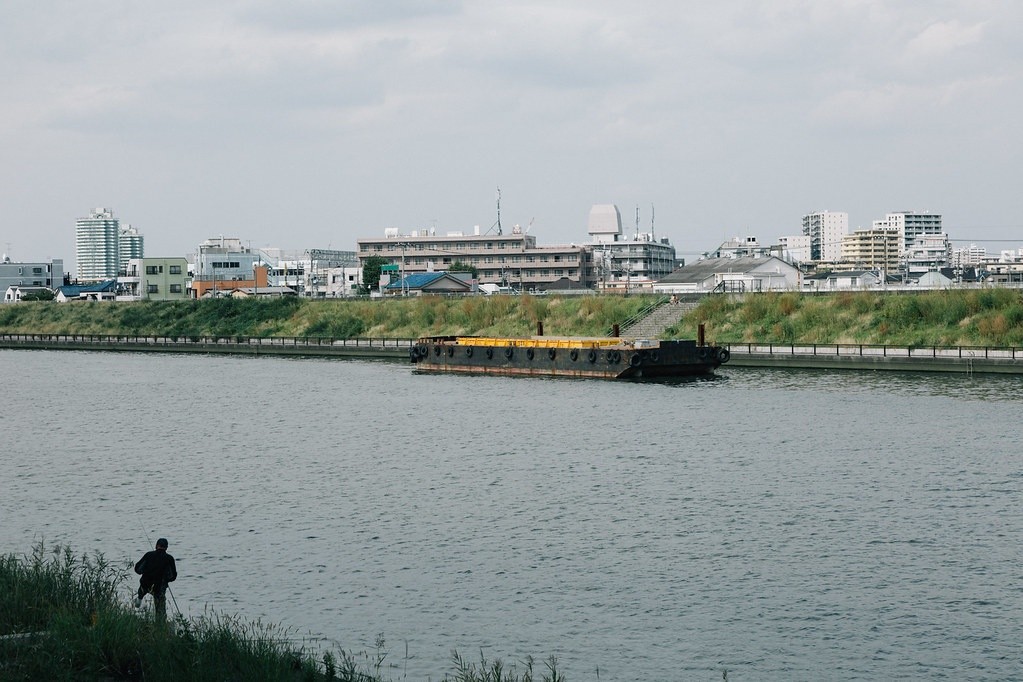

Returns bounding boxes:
[134,538,177,623]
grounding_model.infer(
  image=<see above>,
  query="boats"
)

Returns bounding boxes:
[410,322,729,384]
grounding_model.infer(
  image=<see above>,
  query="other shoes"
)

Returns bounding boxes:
[135,598,140,608]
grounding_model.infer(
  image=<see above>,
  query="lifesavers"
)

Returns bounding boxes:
[465,347,473,357]
[409,345,429,362]
[504,347,513,357]
[434,346,441,356]
[528,347,535,360]
[605,350,621,364]
[588,350,597,362]
[446,346,454,356]
[485,349,493,359]
[548,347,556,360]
[697,346,731,363]
[570,350,579,361]
[630,348,660,366]
